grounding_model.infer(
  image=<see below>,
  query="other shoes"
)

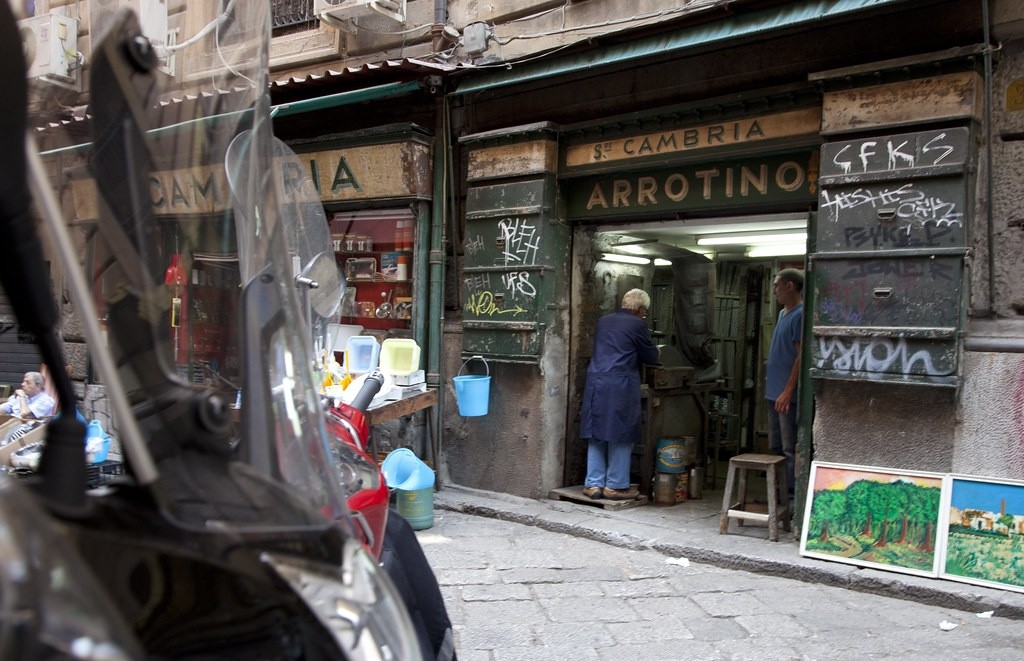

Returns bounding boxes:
[754,496,780,505]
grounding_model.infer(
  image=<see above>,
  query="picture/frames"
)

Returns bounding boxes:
[939,472,1024,595]
[799,461,947,578]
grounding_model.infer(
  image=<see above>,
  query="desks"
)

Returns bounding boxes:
[631,382,718,494]
[365,388,441,495]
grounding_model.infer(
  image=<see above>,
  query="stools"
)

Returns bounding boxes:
[719,453,790,542]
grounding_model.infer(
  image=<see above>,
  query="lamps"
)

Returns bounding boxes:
[696,233,808,245]
[745,246,807,256]
[595,252,651,266]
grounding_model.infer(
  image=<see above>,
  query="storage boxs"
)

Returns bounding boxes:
[86,461,124,487]
[343,335,421,375]
[0,414,49,470]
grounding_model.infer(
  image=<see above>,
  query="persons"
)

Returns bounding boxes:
[763,268,804,499]
[579,289,660,501]
[0,372,55,420]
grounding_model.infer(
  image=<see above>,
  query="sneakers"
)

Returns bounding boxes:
[583,487,602,498]
[602,487,640,500]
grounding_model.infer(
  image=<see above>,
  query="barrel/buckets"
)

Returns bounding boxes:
[655,472,687,507]
[84,420,107,442]
[92,438,111,463]
[381,447,435,490]
[657,437,688,475]
[453,356,491,416]
[688,467,704,499]
[396,488,433,531]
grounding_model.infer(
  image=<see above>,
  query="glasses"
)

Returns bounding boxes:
[642,308,649,319]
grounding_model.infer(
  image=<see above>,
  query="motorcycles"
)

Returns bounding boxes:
[0,1,467,661]
